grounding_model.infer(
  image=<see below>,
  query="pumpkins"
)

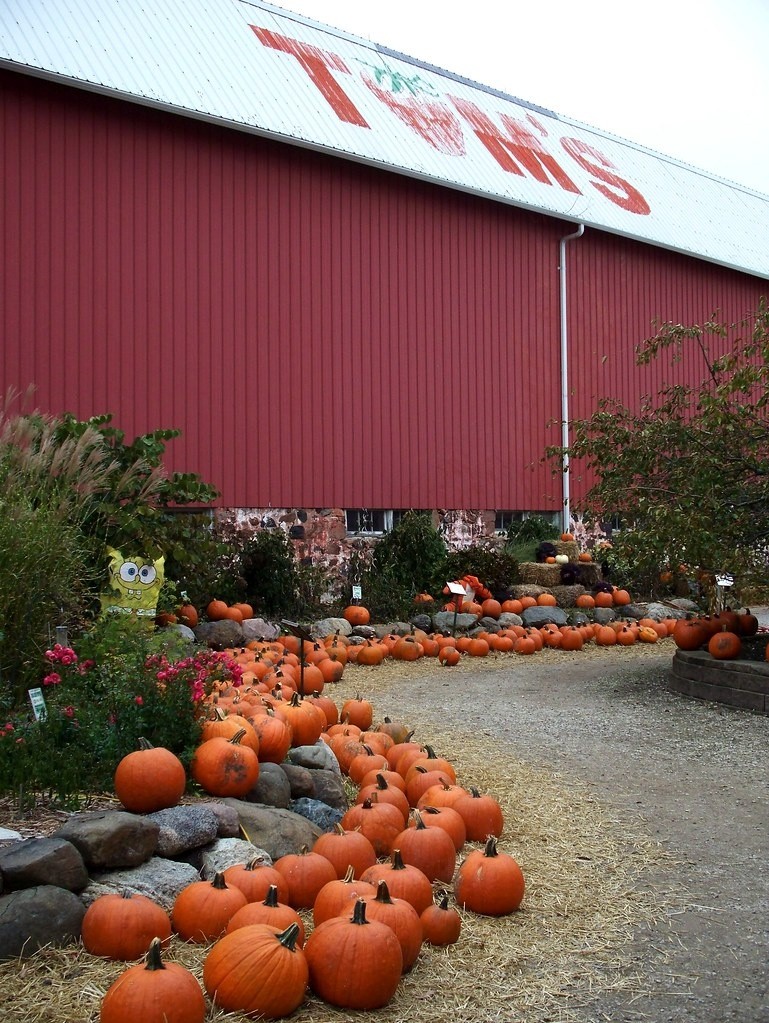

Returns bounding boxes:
[156,526,769,746]
[80,709,525,1023]
